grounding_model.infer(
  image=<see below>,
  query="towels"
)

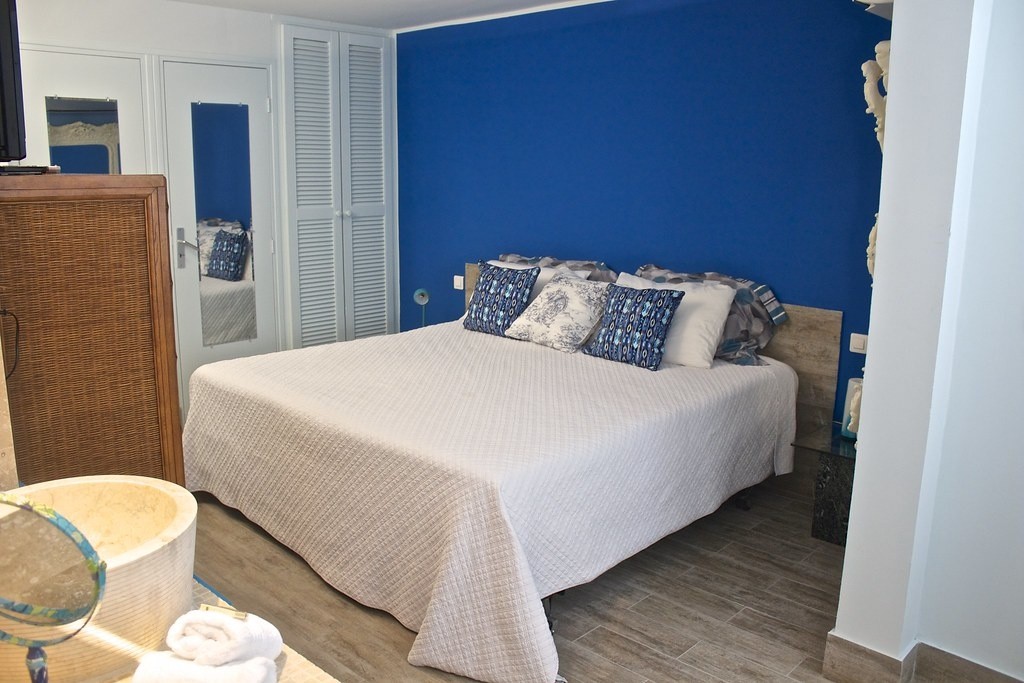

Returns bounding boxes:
[165,608,284,665]
[130,650,278,682]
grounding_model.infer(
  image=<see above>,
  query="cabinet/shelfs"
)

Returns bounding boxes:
[281,22,396,347]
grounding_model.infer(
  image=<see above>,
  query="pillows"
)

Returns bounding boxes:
[198,222,243,276]
[500,254,619,283]
[583,283,684,370]
[616,271,737,370]
[634,266,789,365]
[208,230,250,280]
[464,260,541,338]
[505,264,615,352]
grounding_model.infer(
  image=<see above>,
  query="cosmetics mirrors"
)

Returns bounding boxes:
[0,492,106,683]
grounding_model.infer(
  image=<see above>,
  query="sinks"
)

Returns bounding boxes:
[4,476,198,681]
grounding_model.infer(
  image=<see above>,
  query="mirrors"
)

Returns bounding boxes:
[45,95,122,174]
[190,101,258,346]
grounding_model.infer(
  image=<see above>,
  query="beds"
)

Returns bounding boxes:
[182,263,844,683]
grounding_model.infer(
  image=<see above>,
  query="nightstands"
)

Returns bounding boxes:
[790,422,857,547]
